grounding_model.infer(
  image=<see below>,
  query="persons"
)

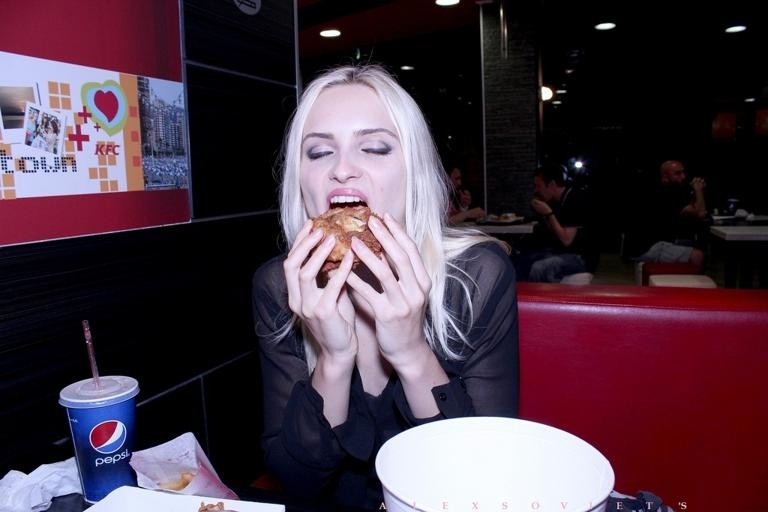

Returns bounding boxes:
[442,164,485,227]
[40,119,58,154]
[527,158,606,284]
[624,159,707,265]
[37,114,49,139]
[249,48,520,510]
[26,108,39,142]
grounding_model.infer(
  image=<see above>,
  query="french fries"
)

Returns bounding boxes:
[156,471,196,489]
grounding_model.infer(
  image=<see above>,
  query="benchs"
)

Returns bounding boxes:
[513,283,768,511]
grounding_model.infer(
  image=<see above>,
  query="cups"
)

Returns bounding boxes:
[728,199,739,212]
[57,377,145,505]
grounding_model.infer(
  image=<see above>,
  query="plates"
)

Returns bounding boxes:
[83,486,287,512]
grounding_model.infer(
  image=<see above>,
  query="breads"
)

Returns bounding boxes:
[499,212,516,221]
[311,203,386,277]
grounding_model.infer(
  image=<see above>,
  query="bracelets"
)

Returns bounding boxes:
[546,211,554,217]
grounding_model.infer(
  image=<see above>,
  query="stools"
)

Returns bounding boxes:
[562,272,593,285]
[641,260,701,287]
[649,274,717,289]
[636,260,645,285]
